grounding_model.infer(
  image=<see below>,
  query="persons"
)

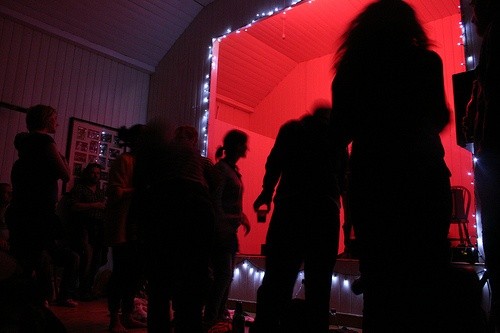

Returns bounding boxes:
[55,120,253,333]
[459,0,500,333]
[0,130,38,301]
[9,104,71,307]
[245,109,361,333]
[331,0,451,333]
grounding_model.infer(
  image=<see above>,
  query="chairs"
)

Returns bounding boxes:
[447,186,474,246]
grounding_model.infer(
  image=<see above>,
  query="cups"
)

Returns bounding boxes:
[255,210,268,222]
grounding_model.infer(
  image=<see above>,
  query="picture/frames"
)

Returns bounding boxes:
[61,117,119,196]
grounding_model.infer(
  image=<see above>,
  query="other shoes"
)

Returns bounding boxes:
[56,297,78,307]
[109,326,124,333]
[120,313,142,328]
[79,292,98,303]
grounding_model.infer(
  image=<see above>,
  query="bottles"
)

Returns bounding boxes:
[329,308,340,329]
[232,301,245,333]
